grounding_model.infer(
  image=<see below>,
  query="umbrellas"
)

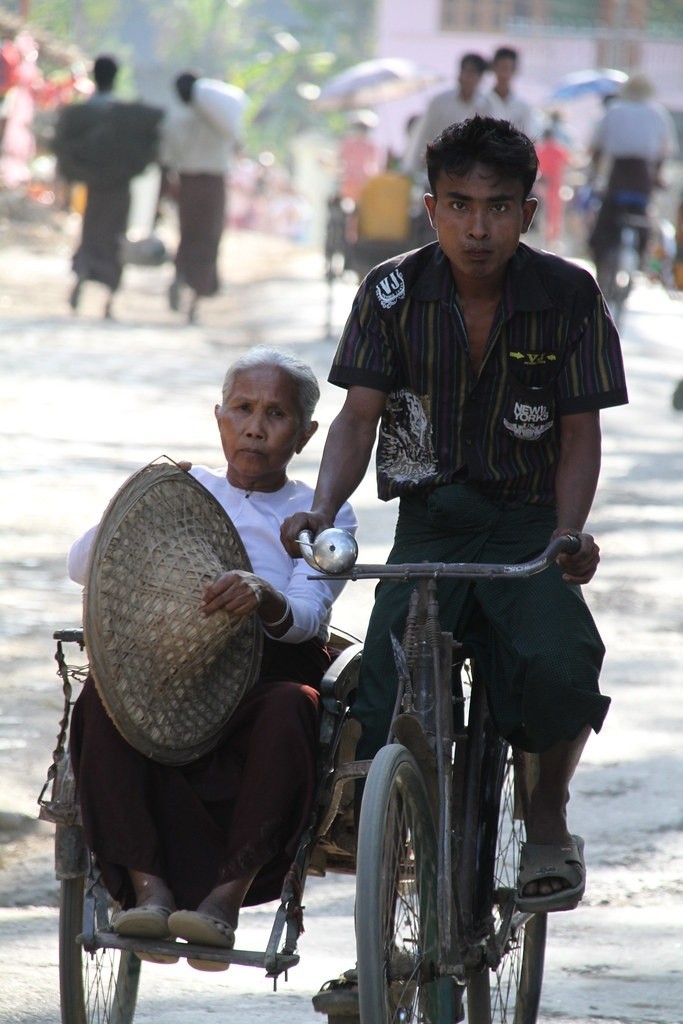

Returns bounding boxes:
[548,67,629,102]
[307,57,442,113]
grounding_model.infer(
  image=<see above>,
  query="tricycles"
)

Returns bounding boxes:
[323,190,439,342]
[41,522,580,1022]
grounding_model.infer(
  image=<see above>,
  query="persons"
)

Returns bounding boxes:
[58,45,671,324]
[279,116,627,1018]
[70,347,358,973]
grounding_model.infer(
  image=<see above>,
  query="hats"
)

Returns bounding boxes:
[87,460,260,761]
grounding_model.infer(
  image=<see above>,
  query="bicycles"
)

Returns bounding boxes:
[578,185,652,330]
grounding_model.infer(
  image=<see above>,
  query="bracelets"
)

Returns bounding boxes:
[262,591,290,627]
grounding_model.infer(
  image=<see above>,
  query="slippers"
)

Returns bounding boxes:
[515,834,584,912]
[309,962,418,1017]
[111,905,239,971]
